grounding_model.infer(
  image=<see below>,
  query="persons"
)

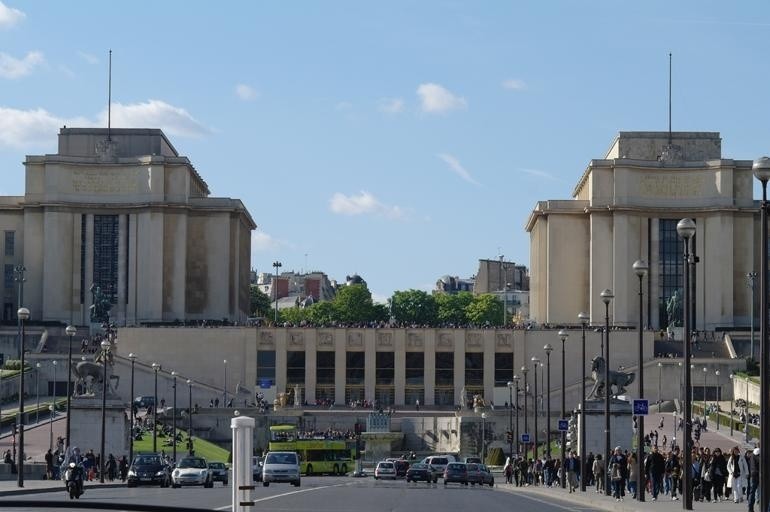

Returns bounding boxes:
[45,449,128,483]
[201,319,653,328]
[315,399,368,408]
[666,290,682,327]
[82,321,118,352]
[504,399,761,510]
[132,398,233,446]
[4,449,18,474]
[659,330,725,358]
[303,430,357,439]
[90,283,107,317]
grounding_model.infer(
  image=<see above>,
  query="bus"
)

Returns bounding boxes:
[269,424,358,477]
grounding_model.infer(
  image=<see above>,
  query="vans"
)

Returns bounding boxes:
[442,462,469,486]
[463,457,482,464]
[253,455,264,480]
[465,462,494,487]
[259,451,301,488]
[420,454,457,477]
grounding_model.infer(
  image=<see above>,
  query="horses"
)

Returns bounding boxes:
[72,350,120,398]
[585,356,636,400]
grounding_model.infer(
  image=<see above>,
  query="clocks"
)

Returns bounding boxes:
[94,138,116,158]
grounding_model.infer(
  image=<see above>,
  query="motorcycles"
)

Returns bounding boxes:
[62,455,90,500]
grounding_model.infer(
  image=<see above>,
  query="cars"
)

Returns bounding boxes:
[170,455,214,488]
[405,463,438,483]
[207,461,230,485]
[126,452,171,488]
[373,457,410,479]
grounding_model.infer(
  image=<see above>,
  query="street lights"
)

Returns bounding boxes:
[507,312,609,491]
[126,352,240,464]
[746,271,758,361]
[14,264,27,360]
[495,254,514,329]
[600,288,614,497]
[751,156,769,512]
[632,258,648,503]
[655,358,752,445]
[34,354,89,456]
[99,338,111,483]
[272,260,282,321]
[479,412,488,463]
[14,306,30,489]
[676,216,696,510]
[62,324,77,446]
